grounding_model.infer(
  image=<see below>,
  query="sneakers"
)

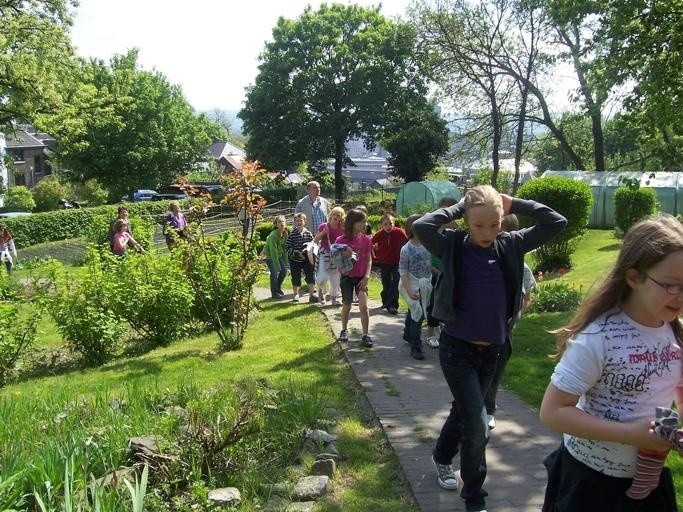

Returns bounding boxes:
[339,328,349,341]
[430,452,460,491]
[271,283,400,315]
[426,335,439,348]
[361,335,374,347]
[403,331,424,360]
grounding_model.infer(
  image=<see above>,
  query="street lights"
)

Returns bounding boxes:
[30,165,34,187]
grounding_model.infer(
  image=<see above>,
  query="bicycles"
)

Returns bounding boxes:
[99,252,148,274]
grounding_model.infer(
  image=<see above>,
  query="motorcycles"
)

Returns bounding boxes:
[58,199,81,209]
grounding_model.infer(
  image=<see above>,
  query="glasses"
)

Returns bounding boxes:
[637,265,683,296]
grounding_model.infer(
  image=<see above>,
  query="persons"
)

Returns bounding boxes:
[0,223,17,275]
[412,186,569,512]
[112,219,146,257]
[133,190,142,201]
[540,215,683,512]
[163,202,189,253]
[109,206,140,255]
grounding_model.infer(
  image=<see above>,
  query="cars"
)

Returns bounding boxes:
[121,183,266,202]
